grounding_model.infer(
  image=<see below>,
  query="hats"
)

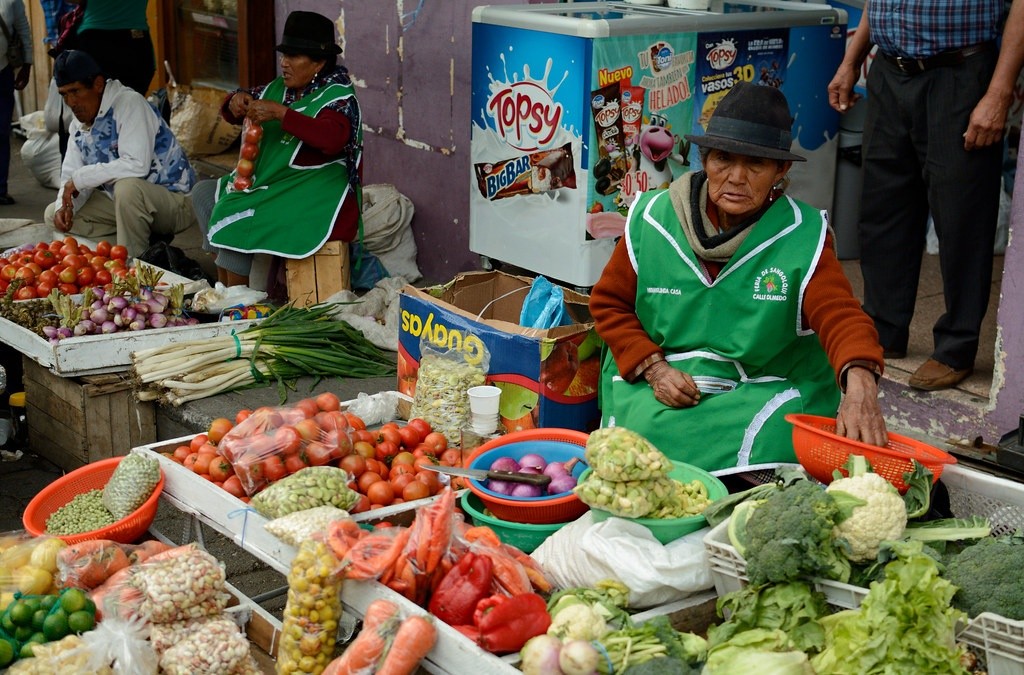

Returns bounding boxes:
[53,49,100,87]
[684,82,807,161]
[273,11,343,56]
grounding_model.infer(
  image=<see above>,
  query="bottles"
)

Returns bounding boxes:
[9,392,28,443]
[460,409,507,465]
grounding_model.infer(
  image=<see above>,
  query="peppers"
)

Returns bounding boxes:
[313,488,554,656]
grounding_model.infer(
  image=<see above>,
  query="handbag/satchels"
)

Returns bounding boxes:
[162,59,244,157]
[7,32,25,68]
[528,509,715,610]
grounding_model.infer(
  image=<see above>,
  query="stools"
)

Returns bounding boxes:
[286,242,351,309]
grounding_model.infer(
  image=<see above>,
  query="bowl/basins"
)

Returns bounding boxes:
[461,490,571,552]
[0,446,45,470]
[577,460,729,546]
[469,440,590,501]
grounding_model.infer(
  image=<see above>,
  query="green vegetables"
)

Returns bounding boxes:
[696,548,977,675]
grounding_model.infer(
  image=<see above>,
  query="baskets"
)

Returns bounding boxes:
[785,414,958,496]
[462,427,591,524]
[702,464,1023,674]
[23,456,165,546]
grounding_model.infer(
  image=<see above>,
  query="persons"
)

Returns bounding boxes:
[191,11,362,299]
[0,0,33,205]
[827,0,1024,390]
[44,50,199,259]
[589,81,889,486]
[41,0,156,174]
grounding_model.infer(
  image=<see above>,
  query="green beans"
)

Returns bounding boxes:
[574,426,713,519]
[101,452,162,524]
[40,487,115,533]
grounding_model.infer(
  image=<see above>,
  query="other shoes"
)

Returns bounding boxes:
[138,232,175,268]
[0,194,15,206]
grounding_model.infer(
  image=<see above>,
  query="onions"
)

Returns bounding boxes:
[42,286,198,342]
[488,453,580,497]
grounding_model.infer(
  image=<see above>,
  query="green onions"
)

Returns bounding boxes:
[120,301,399,407]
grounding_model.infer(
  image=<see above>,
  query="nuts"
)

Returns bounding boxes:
[130,551,266,675]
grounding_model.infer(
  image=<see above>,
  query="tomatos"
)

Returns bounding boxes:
[162,390,468,511]
[234,126,264,190]
[0,237,169,301]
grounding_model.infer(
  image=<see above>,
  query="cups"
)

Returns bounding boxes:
[467,386,502,415]
[0,418,16,444]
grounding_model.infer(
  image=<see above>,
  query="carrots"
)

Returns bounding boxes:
[52,540,203,622]
[322,598,436,675]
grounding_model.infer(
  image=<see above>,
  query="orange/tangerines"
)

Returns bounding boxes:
[0,533,96,673]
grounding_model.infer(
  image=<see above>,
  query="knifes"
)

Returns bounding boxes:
[418,465,551,485]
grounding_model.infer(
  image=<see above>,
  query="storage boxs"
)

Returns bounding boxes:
[399,271,603,436]
[22,363,159,468]
[704,461,1024,675]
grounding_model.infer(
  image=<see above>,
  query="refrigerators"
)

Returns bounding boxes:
[470,0,847,297]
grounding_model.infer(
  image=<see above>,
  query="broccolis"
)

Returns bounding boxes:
[726,452,1024,624]
[616,615,711,675]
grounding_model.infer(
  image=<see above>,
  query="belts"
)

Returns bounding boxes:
[880,41,991,76]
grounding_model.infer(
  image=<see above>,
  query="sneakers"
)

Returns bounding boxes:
[908,358,974,390]
[883,349,907,359]
